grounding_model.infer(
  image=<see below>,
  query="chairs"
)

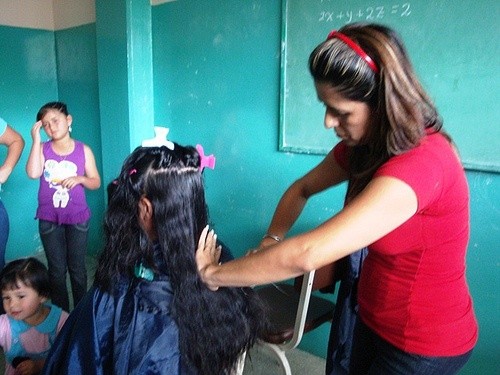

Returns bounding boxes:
[255,261,341,375]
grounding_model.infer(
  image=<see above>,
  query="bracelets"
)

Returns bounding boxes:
[262,234,282,243]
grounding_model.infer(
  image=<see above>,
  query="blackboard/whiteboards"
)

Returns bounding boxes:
[277,1,500,174]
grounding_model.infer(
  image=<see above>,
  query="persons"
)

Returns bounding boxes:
[196,20,478,375]
[42,126,270,375]
[0,118,25,266]
[0,257,70,375]
[26,102,101,311]
